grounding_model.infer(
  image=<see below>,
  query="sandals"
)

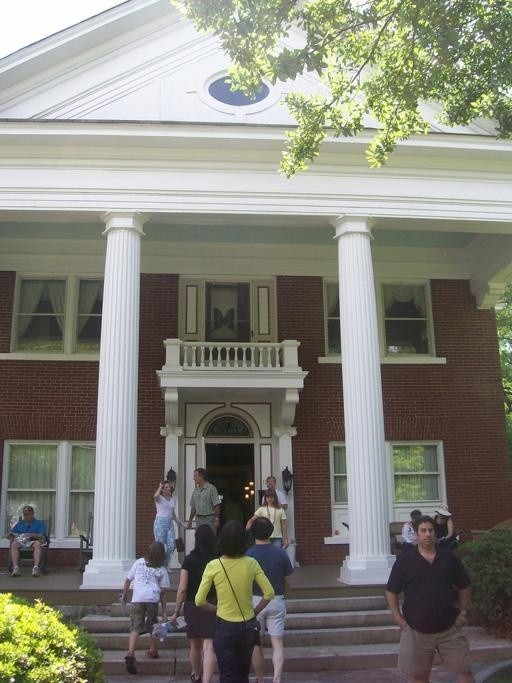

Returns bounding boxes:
[125,653,137,674]
[147,648,158,658]
[191,673,201,683]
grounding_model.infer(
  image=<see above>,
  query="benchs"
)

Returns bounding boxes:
[339,517,462,560]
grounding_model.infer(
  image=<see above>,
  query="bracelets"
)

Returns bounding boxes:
[215,517,220,519]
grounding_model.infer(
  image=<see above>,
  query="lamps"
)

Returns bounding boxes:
[280,465,294,496]
[165,466,176,498]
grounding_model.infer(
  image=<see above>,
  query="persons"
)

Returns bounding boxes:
[401,510,422,550]
[119,542,170,676]
[188,468,221,538]
[384,515,478,683]
[152,480,185,572]
[173,524,223,682]
[194,520,274,683]
[433,503,454,548]
[9,505,47,577]
[245,489,289,549]
[244,517,294,683]
[261,476,288,510]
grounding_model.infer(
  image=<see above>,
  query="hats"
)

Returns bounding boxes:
[22,507,34,514]
[435,504,452,516]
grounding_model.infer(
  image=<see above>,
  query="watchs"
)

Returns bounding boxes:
[459,607,468,618]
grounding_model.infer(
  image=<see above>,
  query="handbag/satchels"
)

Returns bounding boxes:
[246,621,260,645]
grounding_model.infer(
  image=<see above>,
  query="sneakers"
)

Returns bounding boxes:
[11,566,40,577]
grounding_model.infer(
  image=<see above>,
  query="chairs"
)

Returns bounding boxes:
[6,513,55,573]
[77,510,96,574]
